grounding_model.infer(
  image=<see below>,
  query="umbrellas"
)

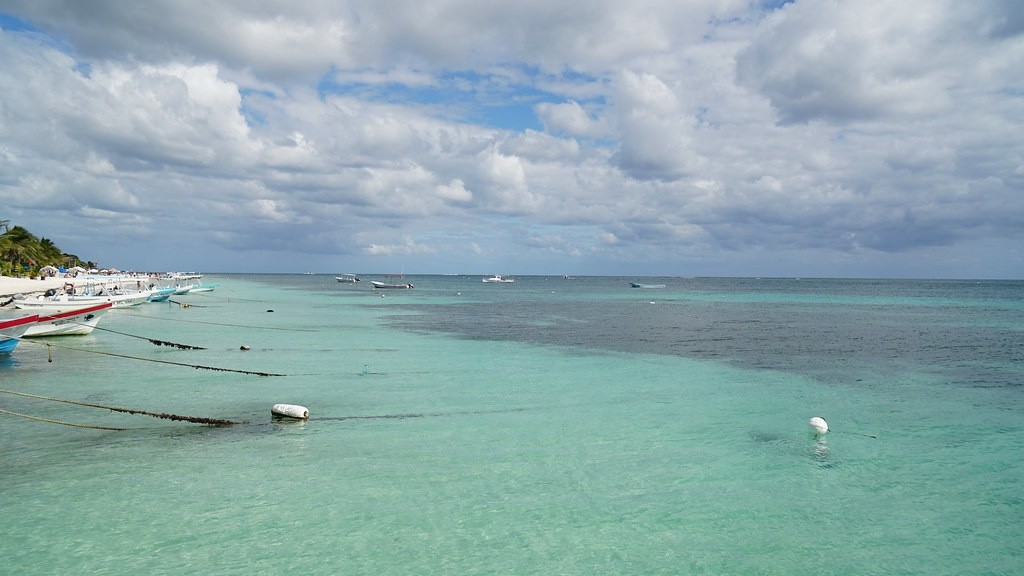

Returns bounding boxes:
[40,265,121,276]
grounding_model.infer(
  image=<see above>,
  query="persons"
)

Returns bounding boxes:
[149,282,154,287]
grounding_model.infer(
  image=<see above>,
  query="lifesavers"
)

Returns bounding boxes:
[64,282,74,291]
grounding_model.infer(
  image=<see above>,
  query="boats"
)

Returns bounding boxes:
[629,281,666,289]
[334,274,360,283]
[0,272,219,354]
[371,281,413,289]
[562,275,575,279]
[482,275,514,283]
